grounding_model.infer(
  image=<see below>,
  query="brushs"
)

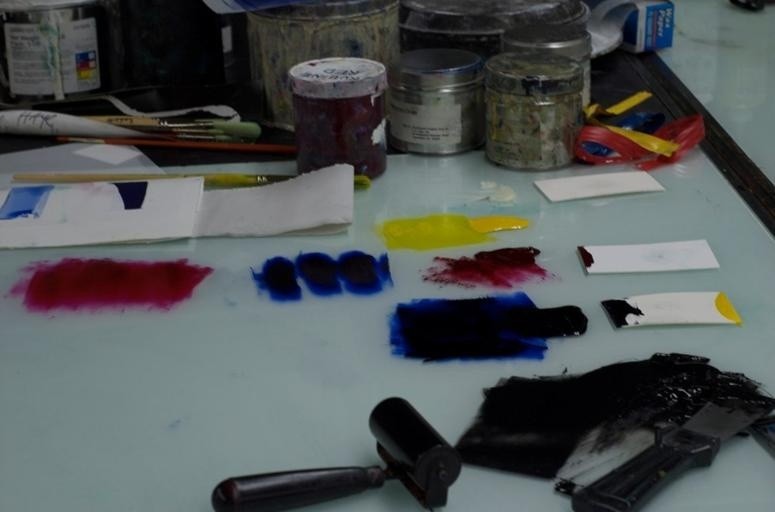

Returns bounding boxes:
[212,397,461,512]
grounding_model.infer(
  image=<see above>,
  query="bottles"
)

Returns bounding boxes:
[290,55,399,184]
[502,25,596,105]
[388,50,490,153]
[1,1,111,100]
[484,54,587,168]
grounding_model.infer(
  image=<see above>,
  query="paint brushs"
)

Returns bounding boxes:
[11,174,371,192]
[56,115,296,154]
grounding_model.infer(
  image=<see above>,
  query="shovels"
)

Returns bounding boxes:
[571,393,775,511]
